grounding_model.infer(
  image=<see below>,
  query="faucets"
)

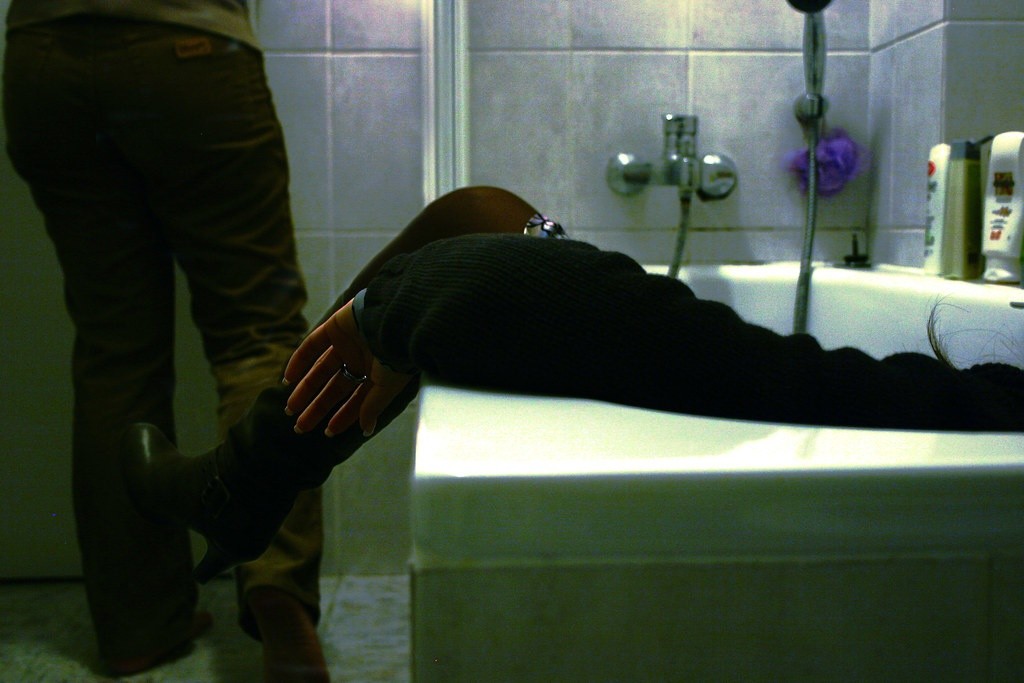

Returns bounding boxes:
[658,113,701,192]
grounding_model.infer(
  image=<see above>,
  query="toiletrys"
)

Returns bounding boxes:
[922,129,1024,284]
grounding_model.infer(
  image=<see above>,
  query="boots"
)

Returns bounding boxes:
[117,292,418,585]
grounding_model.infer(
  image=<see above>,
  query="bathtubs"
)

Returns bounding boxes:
[406,257,1023,682]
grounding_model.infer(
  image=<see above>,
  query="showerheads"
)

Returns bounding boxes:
[783,1,836,123]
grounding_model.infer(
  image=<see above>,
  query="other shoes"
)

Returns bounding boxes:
[241,587,329,683]
[102,611,212,677]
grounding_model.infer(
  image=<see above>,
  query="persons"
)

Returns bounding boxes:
[0,0,340,683]
[120,186,1024,591]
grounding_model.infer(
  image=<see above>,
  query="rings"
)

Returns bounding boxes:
[341,363,366,384]
